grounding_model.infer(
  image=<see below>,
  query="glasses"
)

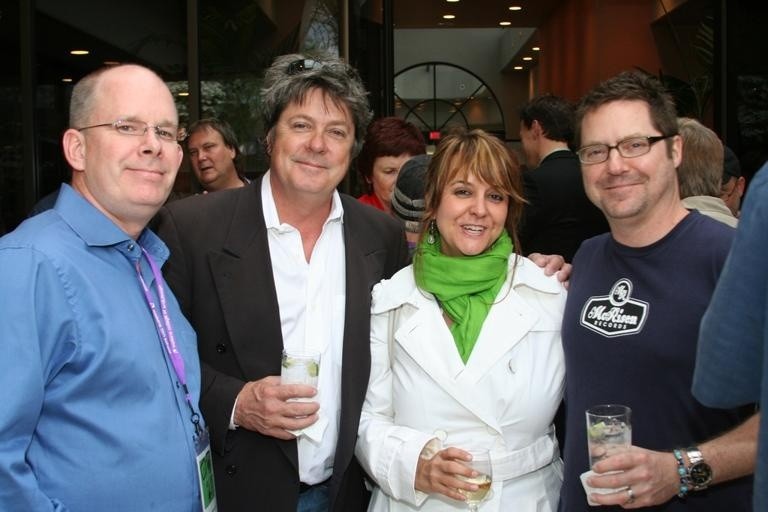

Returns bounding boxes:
[575,134,680,165]
[77,119,180,141]
[285,58,356,76]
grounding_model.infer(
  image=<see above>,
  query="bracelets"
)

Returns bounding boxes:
[671,449,689,499]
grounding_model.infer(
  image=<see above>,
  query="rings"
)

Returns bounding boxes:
[628,486,635,499]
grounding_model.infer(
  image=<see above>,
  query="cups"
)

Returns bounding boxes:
[586,405,633,471]
[280,349,322,436]
[455,448,492,512]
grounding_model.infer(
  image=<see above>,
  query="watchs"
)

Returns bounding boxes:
[688,445,713,492]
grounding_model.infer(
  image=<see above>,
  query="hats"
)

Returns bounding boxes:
[389,154,436,233]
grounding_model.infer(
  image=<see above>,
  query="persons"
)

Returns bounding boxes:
[355,130,568,511]
[148,51,572,512]
[352,118,429,217]
[1,64,216,511]
[555,68,761,512]
[692,163,767,510]
[673,114,740,231]
[391,155,432,260]
[512,92,610,267]
[715,146,745,217]
[182,114,257,197]
